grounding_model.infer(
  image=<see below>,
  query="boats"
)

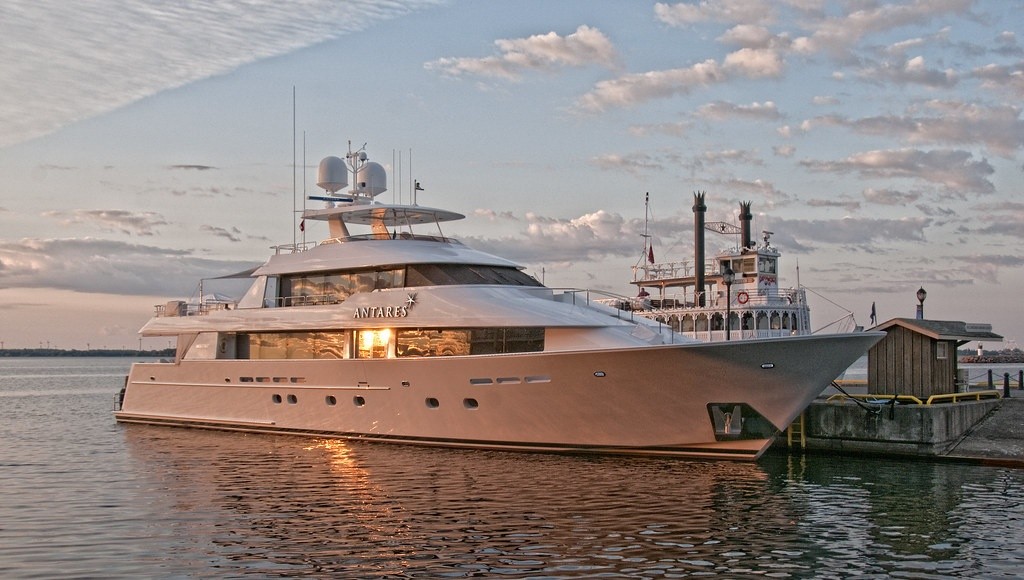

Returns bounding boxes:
[108,83,888,464]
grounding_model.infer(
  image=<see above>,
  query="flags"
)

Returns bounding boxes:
[416,183,424,190]
[299,220,304,231]
[870,303,875,326]
[648,243,654,264]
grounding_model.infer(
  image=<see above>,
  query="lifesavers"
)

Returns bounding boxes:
[737,292,750,305]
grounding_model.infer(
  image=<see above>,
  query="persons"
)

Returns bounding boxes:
[742,321,748,330]
[225,304,231,310]
[715,321,721,330]
[722,326,724,330]
[637,287,650,297]
